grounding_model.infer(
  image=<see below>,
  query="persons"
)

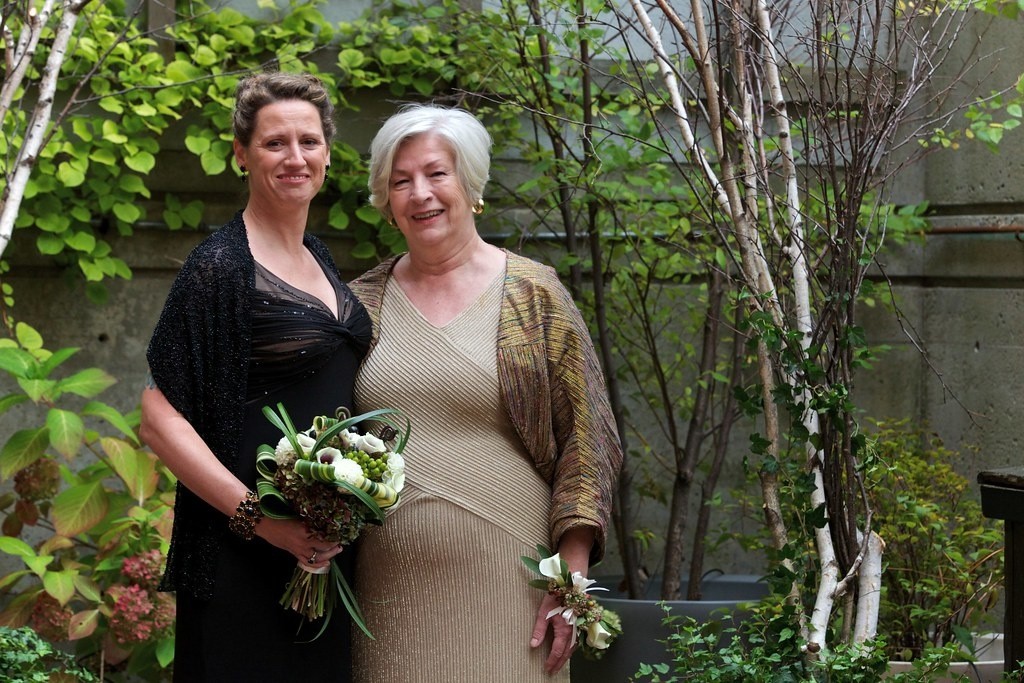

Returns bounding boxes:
[137,73,374,683]
[347,104,622,683]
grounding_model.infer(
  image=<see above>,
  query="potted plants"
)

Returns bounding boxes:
[841,415,1005,683]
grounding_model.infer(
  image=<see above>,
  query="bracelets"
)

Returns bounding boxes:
[517,543,622,659]
[226,491,263,540]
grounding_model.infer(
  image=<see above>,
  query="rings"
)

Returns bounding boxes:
[304,552,320,564]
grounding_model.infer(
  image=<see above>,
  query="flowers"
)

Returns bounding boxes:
[257,402,411,643]
